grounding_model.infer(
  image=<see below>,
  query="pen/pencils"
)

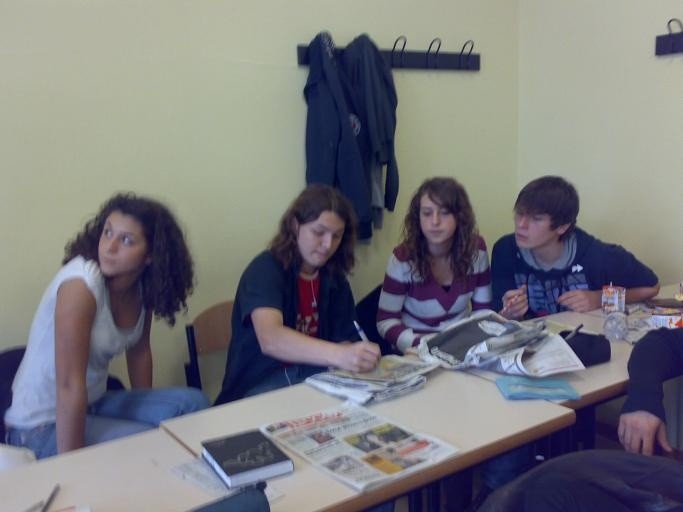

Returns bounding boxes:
[563,323,584,341]
[497,294,520,315]
[23,483,60,511]
[353,320,370,342]
[625,304,639,315]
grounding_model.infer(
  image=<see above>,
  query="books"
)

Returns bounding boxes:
[201,430,294,490]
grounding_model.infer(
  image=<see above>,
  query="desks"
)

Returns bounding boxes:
[1,284,682,510]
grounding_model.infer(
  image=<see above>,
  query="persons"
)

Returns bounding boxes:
[478,326,683,512]
[4,193,211,458]
[376,177,493,354]
[213,183,382,405]
[491,176,660,320]
